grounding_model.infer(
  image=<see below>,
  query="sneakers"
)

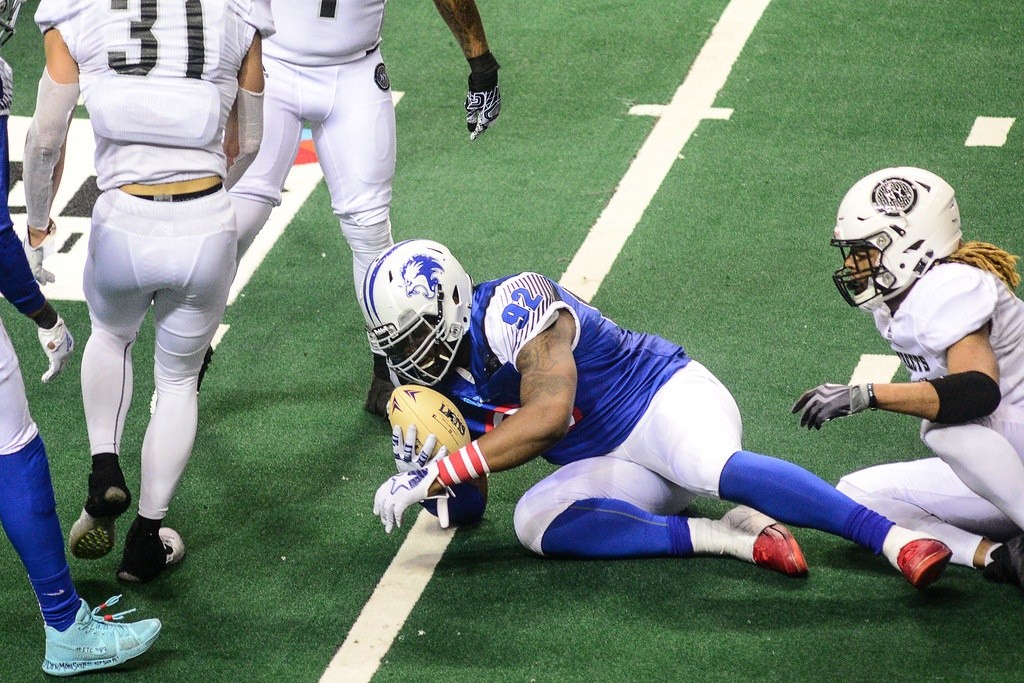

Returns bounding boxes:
[117,527,186,582]
[42,598,162,676]
[364,376,396,421]
[984,535,1024,593]
[69,471,131,559]
[716,504,809,580]
[897,538,953,589]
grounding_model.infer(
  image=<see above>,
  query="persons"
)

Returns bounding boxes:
[791,166,1024,592]
[25,0,275,590]
[195,0,501,421]
[360,239,952,588]
[0,0,162,678]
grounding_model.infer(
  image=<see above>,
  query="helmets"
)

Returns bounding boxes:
[830,166,962,312]
[360,239,473,384]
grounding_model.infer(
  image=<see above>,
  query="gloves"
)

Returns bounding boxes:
[463,51,502,141]
[37,314,75,384]
[23,219,56,287]
[392,424,448,473]
[373,463,456,534]
[792,383,877,431]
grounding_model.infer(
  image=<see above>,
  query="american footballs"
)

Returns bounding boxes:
[387,384,471,461]
